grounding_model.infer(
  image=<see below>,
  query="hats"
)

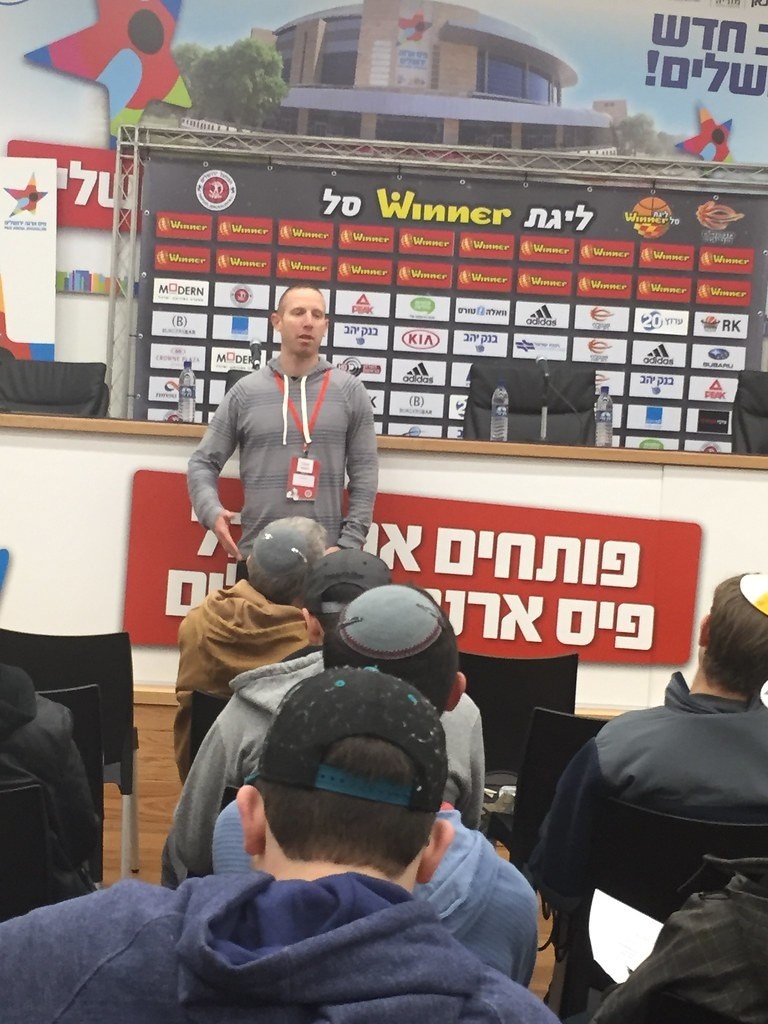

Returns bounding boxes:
[254,526,306,577]
[242,666,447,811]
[740,573,768,616]
[303,548,390,615]
[337,583,443,659]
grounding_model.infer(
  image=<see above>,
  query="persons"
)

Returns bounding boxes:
[0,663,101,922]
[0,667,563,1024]
[161,516,768,1024]
[187,283,379,584]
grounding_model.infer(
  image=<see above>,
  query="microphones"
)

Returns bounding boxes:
[250,339,260,369]
[536,355,550,378]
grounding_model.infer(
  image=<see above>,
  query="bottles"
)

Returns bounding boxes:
[489,380,508,442]
[177,362,196,423]
[595,386,612,447]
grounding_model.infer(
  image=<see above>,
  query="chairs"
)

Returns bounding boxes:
[462,358,596,446]
[731,370,767,456]
[0,628,139,924]
[457,650,768,1024]
[0,359,109,418]
